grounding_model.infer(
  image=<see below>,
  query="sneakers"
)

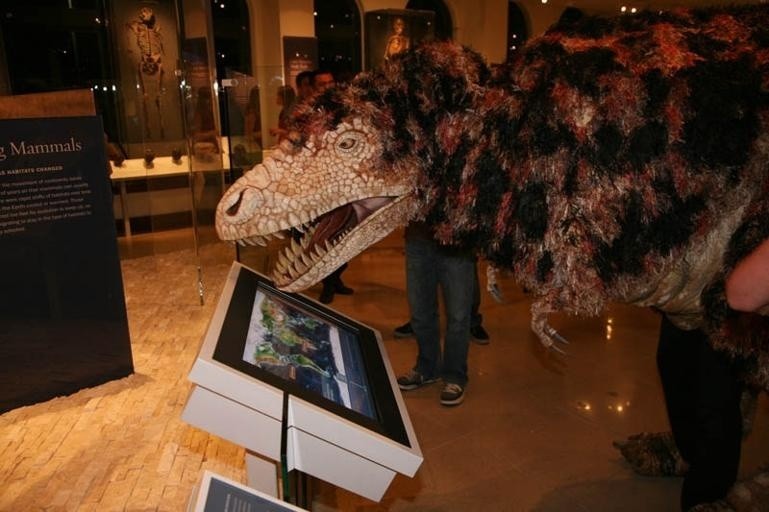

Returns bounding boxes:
[397,369,441,390]
[394,321,414,338]
[441,383,465,404]
[471,324,489,344]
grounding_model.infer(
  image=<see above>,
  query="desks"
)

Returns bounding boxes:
[108,153,231,239]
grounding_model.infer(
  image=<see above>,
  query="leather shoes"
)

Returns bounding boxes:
[320,284,334,303]
[337,278,353,294]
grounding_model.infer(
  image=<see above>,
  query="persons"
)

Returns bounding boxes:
[658,217,768,512]
[389,47,498,348]
[396,2,475,406]
[268,84,296,144]
[295,72,316,98]
[193,87,224,188]
[244,85,262,149]
[298,68,356,306]
[220,83,244,137]
